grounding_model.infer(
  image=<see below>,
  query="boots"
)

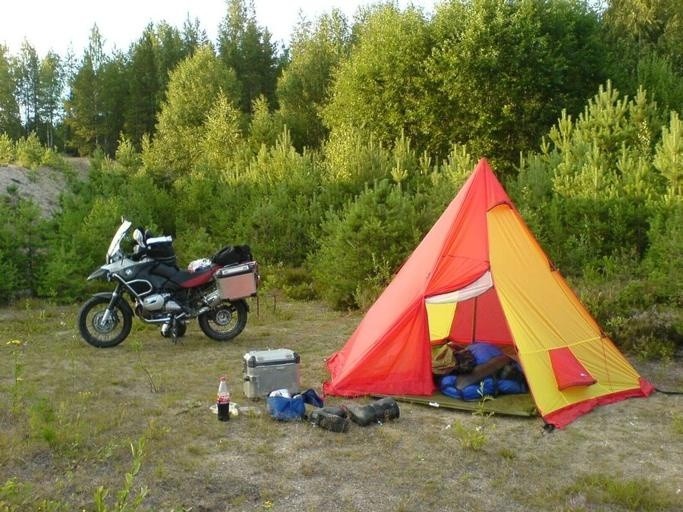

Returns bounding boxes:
[341,396,401,427]
[310,403,349,434]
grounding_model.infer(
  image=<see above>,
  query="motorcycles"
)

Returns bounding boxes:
[77,217,261,348]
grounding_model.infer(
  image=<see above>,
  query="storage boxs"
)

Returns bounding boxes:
[241,348,301,399]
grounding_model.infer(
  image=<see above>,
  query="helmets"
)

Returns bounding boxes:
[160,320,187,339]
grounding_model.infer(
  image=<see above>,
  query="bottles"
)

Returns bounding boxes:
[242,376,260,401]
[216,378,231,421]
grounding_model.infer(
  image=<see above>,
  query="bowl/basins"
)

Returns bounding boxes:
[209,401,236,414]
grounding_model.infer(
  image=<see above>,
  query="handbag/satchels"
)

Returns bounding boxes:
[265,389,307,421]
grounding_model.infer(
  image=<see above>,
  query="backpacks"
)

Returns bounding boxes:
[210,243,253,267]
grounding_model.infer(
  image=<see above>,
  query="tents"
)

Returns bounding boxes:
[320,156,659,432]
